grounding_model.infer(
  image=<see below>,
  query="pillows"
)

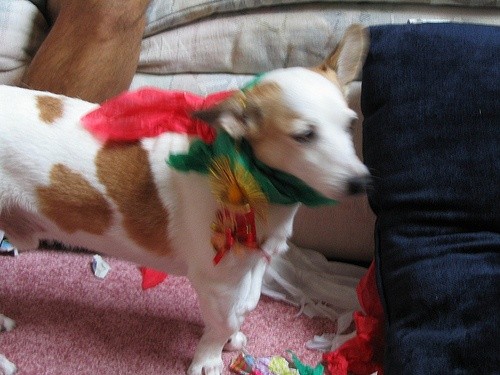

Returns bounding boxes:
[359,23,499,374]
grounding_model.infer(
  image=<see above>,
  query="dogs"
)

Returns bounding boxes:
[1,22,374,375]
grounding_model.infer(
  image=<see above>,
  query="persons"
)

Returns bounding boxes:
[18,0,156,111]
